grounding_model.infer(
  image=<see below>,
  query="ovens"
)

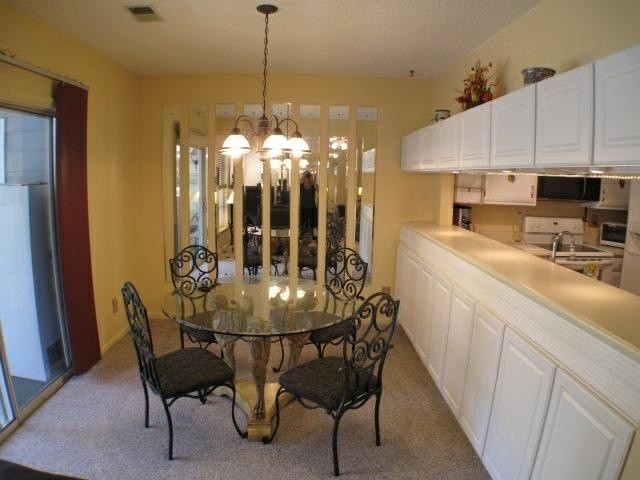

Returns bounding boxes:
[555,256,614,288]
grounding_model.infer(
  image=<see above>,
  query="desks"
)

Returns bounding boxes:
[160,278,363,442]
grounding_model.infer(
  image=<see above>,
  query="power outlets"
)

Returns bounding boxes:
[112,297,119,313]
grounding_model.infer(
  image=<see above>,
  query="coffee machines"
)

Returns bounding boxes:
[452,205,474,234]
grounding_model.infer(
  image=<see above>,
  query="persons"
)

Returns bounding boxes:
[299,174,319,242]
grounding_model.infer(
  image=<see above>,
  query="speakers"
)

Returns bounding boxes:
[244,185,258,225]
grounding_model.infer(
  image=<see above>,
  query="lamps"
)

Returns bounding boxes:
[222,3,311,160]
[270,160,310,168]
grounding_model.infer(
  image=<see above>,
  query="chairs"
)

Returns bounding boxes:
[259,293,401,477]
[241,217,347,277]
[272,246,368,384]
[169,245,251,394]
[121,281,247,461]
[0,183,66,380]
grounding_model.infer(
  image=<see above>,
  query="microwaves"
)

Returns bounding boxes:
[538,176,602,203]
[599,221,628,248]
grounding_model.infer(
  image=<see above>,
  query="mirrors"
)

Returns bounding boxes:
[162,102,374,289]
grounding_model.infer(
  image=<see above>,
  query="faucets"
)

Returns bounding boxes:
[547,230,576,262]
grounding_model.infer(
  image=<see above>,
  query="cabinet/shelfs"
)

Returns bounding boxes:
[402,43,640,180]
[396,220,639,480]
